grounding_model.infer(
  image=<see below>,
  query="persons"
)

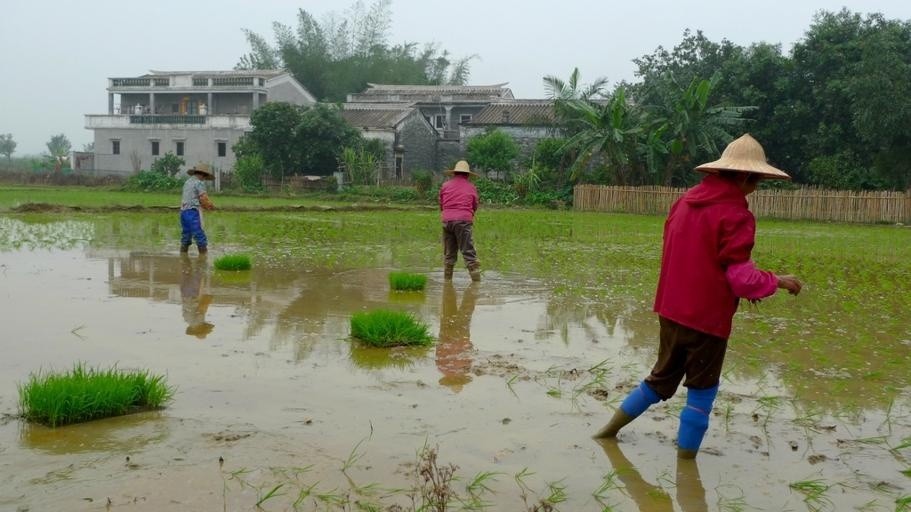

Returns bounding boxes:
[591,131,805,459]
[436,160,484,282]
[179,164,217,253]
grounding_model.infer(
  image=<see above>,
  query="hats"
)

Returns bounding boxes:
[443,161,480,176]
[694,132,790,179]
[186,163,214,180]
